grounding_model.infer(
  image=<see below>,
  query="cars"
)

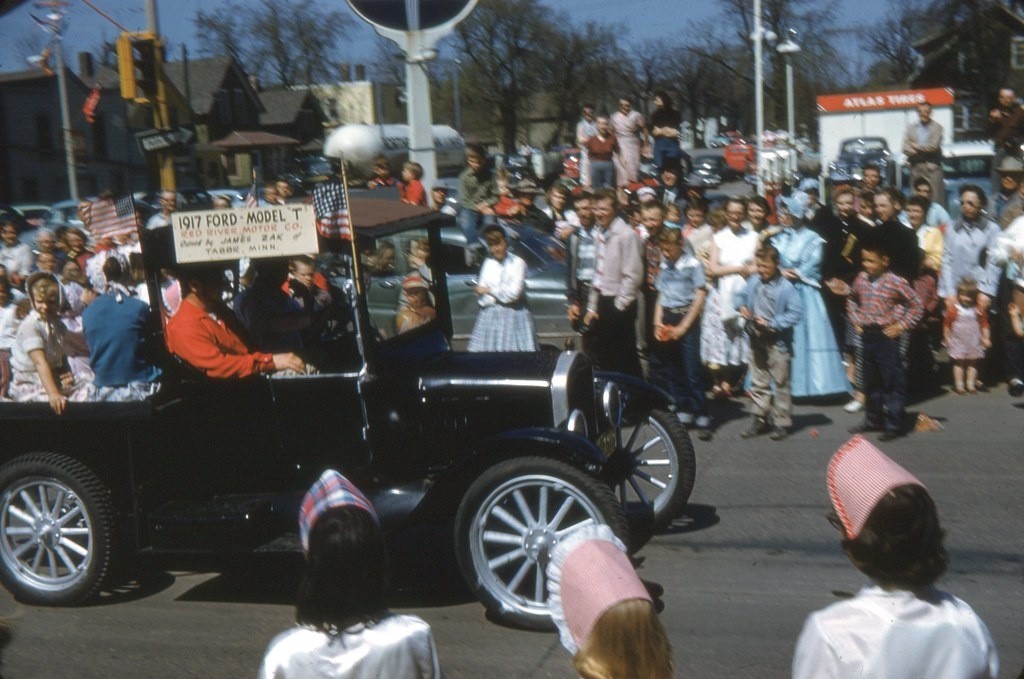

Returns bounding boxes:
[9,188,270,229]
[0,130,698,633]
[938,141,996,226]
[824,137,892,183]
[683,142,756,188]
[560,145,584,182]
[331,219,649,359]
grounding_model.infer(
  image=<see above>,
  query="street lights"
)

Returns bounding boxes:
[777,27,801,147]
[748,0,777,200]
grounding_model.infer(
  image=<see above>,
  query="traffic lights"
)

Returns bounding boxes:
[130,39,166,105]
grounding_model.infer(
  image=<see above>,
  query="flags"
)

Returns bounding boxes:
[246,181,259,207]
[313,182,352,241]
[80,193,138,237]
[83,88,101,123]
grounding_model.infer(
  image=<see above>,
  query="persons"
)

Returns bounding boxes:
[0,89,1024,440]
[256,469,443,679]
[546,524,677,679]
[791,433,1001,678]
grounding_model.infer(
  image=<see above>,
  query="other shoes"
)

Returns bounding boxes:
[844,400,865,412]
[954,381,987,394]
[711,381,731,399]
[730,380,743,394]
[698,423,712,439]
[741,415,769,438]
[1009,382,1024,396]
[879,429,906,441]
[770,427,793,440]
[847,419,875,434]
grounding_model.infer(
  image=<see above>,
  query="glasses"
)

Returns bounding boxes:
[827,511,846,532]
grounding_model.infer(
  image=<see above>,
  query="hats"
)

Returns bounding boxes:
[478,223,510,256]
[25,270,64,312]
[800,177,819,192]
[774,193,804,219]
[86,252,131,295]
[546,524,656,656]
[517,179,545,194]
[404,276,430,290]
[996,156,1024,173]
[913,175,934,202]
[827,432,927,542]
[298,470,380,561]
